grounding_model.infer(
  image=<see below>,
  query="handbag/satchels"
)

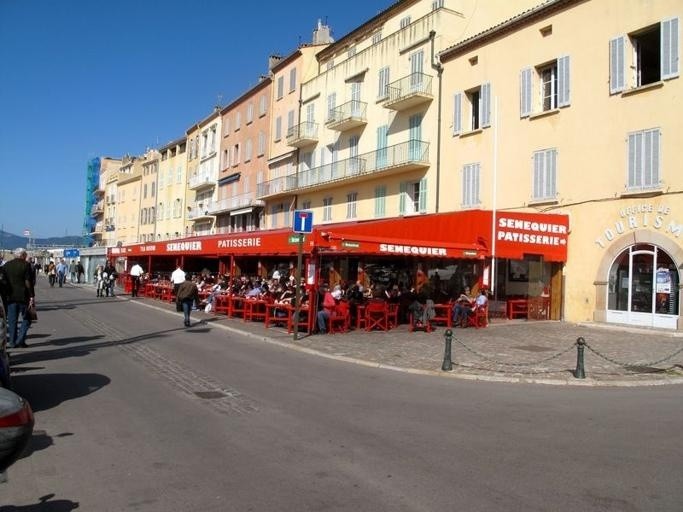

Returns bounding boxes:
[24,309,37,320]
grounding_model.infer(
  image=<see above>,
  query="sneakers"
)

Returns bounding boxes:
[10,342,28,348]
[184,321,190,326]
[453,321,459,326]
[463,322,467,327]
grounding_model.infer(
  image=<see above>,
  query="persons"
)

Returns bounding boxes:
[93,261,489,337]
[0,246,85,387]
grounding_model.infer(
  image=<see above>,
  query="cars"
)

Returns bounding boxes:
[0,386,35,475]
